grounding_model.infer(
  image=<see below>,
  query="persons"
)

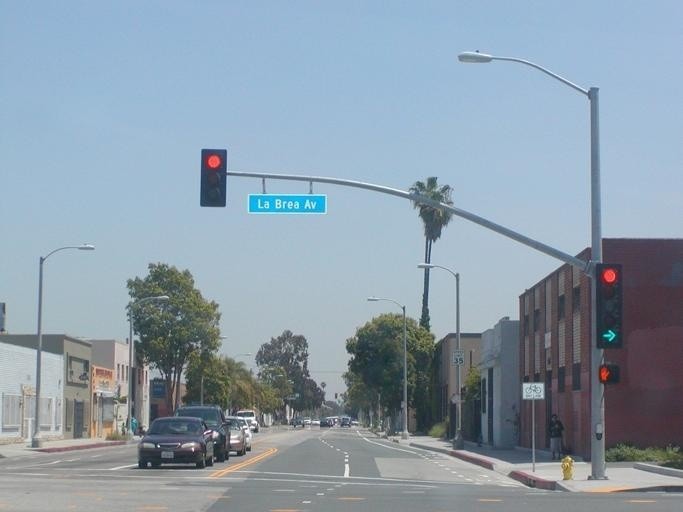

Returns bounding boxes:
[137,421,147,438]
[130,415,137,434]
[546,413,564,461]
[120,422,126,435]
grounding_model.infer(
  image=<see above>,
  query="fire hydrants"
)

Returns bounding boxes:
[561,456,574,481]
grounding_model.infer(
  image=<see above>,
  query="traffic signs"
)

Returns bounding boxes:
[452,350,465,365]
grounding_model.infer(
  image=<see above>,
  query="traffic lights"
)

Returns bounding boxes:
[595,263,622,383]
[200,148,227,207]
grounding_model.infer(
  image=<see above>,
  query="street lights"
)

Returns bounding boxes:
[257,367,295,421]
[232,352,252,359]
[459,50,609,482]
[201,335,228,406]
[367,297,409,439]
[126,294,169,437]
[32,244,95,449]
[418,263,463,450]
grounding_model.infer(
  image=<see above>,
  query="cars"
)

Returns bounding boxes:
[138,406,260,468]
[293,415,359,428]
